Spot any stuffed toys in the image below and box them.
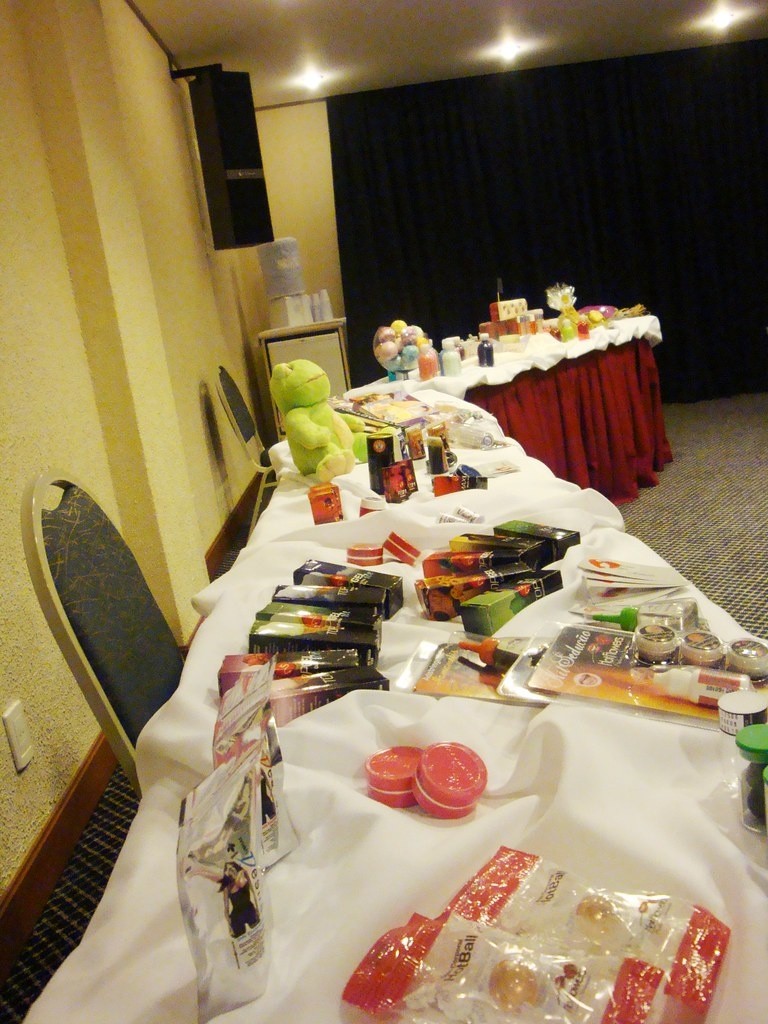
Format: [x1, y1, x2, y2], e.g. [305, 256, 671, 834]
[269, 359, 403, 484]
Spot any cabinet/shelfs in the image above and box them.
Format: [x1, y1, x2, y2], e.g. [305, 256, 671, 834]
[259, 321, 350, 442]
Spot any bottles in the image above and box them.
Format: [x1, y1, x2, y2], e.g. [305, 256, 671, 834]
[560, 319, 575, 343]
[360, 497, 383, 517]
[591, 597, 698, 633]
[418, 336, 465, 382]
[633, 626, 768, 690]
[477, 332, 495, 367]
[735, 724, 768, 838]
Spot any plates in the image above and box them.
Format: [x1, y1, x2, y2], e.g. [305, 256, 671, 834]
[578, 306, 616, 318]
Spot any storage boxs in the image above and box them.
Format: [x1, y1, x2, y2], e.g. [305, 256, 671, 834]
[218, 520, 580, 727]
[269, 295, 314, 329]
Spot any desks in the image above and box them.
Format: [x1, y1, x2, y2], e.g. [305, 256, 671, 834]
[23, 315, 768, 1024]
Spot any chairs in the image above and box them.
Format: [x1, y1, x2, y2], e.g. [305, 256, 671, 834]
[215, 366, 277, 543]
[23, 468, 185, 805]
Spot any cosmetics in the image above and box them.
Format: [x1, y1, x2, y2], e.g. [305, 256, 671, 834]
[308, 482, 343, 525]
[417, 332, 496, 382]
[360, 422, 488, 517]
[479, 297, 544, 344]
[413, 520, 581, 641]
[218, 558, 404, 726]
[363, 740, 489, 819]
[346, 530, 422, 567]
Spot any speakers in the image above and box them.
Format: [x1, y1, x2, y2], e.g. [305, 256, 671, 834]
[188, 70, 275, 250]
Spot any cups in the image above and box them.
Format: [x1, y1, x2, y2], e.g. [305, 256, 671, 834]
[366, 433, 394, 495]
[393, 460, 418, 492]
[381, 465, 410, 504]
